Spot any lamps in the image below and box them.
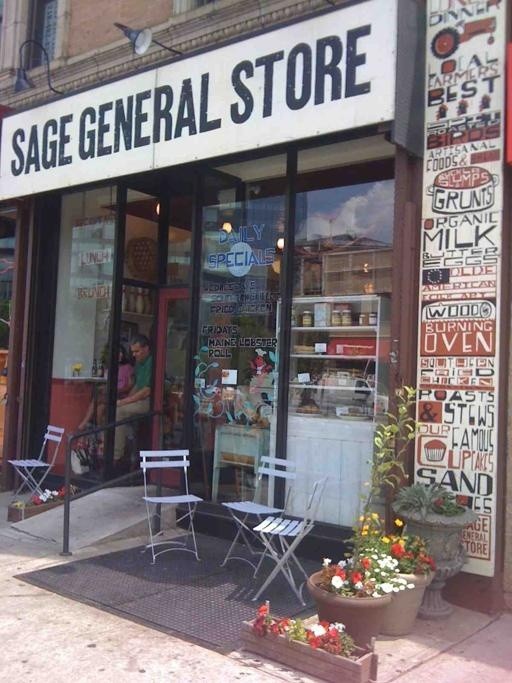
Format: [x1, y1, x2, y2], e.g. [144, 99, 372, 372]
[13, 39, 65, 96]
[112, 21, 183, 57]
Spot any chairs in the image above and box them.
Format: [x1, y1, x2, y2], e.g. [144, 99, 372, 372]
[139, 450, 204, 563]
[218, 456, 295, 579]
[251, 476, 328, 606]
[6, 424, 65, 502]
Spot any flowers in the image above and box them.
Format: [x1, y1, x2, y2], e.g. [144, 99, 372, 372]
[12, 483, 79, 508]
[321, 547, 415, 597]
[346, 513, 438, 574]
[249, 600, 357, 657]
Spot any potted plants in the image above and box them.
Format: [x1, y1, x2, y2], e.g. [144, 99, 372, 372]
[390, 480, 478, 620]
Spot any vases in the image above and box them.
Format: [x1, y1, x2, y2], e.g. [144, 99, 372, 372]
[380, 573, 434, 636]
[6, 501, 62, 522]
[308, 571, 392, 647]
[240, 614, 376, 682]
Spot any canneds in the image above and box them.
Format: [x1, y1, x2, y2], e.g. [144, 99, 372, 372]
[342, 310, 353, 326]
[358, 314, 366, 325]
[368, 313, 377, 326]
[331, 310, 342, 326]
[302, 310, 313, 327]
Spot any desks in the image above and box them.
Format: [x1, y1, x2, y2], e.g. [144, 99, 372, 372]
[211, 424, 270, 505]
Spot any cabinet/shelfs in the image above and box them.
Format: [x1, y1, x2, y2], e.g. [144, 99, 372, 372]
[292, 247, 392, 295]
[267, 296, 391, 531]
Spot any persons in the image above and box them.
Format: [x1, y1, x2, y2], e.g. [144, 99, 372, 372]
[74, 344, 134, 432]
[113, 333, 155, 461]
